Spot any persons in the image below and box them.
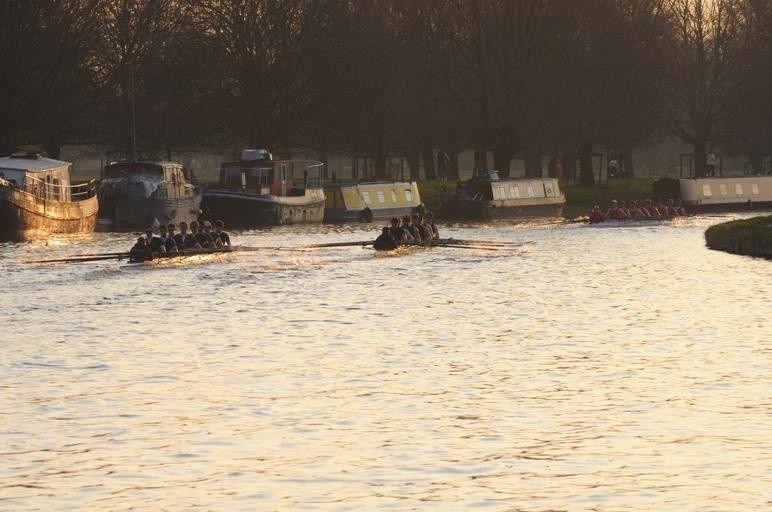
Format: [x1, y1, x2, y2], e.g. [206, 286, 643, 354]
[144, 220, 230, 254]
[390, 212, 439, 245]
[604, 199, 684, 219]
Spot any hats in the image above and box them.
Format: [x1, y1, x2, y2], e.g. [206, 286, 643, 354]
[145, 226, 153, 232]
[204, 221, 211, 226]
[215, 222, 223, 226]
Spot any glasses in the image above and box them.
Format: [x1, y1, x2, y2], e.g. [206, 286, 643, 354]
[168, 229, 173, 231]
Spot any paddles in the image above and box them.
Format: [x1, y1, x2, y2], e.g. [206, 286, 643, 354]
[22, 252, 129, 264]
[221, 239, 376, 252]
[412, 237, 514, 251]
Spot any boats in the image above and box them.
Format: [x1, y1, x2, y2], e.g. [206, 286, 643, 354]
[200, 148, 328, 225]
[0, 145, 100, 247]
[119, 249, 233, 268]
[578, 216, 683, 228]
[324, 180, 434, 222]
[94, 155, 203, 232]
[372, 234, 440, 258]
[447, 170, 567, 221]
